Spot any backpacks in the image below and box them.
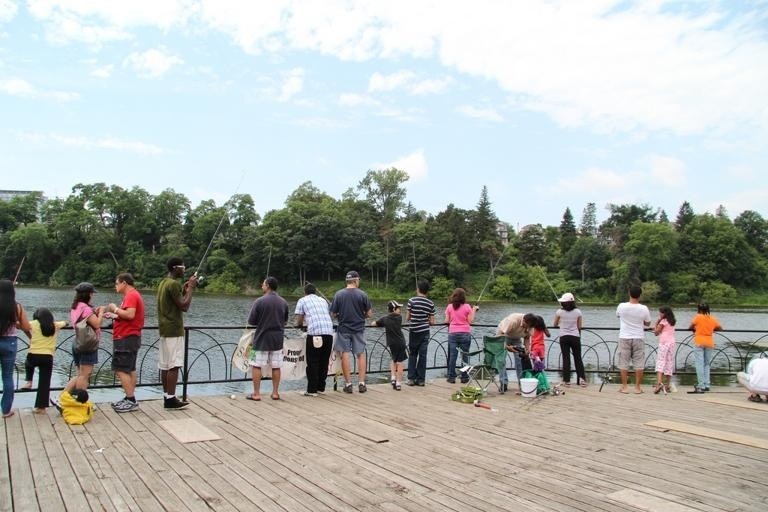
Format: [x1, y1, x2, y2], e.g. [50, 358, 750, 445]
[72, 305, 99, 355]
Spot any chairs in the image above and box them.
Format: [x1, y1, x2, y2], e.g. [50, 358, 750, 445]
[457, 335, 507, 397]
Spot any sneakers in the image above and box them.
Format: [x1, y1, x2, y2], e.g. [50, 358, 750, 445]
[1, 410, 15, 417]
[21, 382, 32, 389]
[653, 383, 672, 394]
[343, 383, 352, 394]
[446, 378, 455, 383]
[580, 381, 587, 387]
[73, 280, 99, 295]
[303, 390, 317, 397]
[317, 388, 327, 395]
[357, 383, 367, 392]
[694, 386, 710, 394]
[748, 395, 762, 402]
[390, 379, 402, 391]
[564, 382, 571, 388]
[163, 396, 190, 409]
[405, 380, 424, 387]
[32, 407, 48, 415]
[462, 375, 469, 383]
[110, 398, 139, 413]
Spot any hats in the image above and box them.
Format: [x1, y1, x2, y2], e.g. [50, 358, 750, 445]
[389, 301, 403, 310]
[557, 293, 575, 303]
[345, 271, 360, 281]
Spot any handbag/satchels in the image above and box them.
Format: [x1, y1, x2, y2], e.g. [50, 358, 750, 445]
[519, 368, 551, 395]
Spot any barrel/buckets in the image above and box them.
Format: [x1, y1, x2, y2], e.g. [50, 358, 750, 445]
[519, 378, 539, 398]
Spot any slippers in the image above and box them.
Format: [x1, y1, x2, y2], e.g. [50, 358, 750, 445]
[270, 393, 279, 400]
[246, 394, 260, 401]
[619, 387, 644, 394]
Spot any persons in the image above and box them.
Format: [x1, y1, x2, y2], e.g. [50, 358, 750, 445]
[0, 258, 200, 418]
[495, 285, 768, 403]
[246, 271, 479, 401]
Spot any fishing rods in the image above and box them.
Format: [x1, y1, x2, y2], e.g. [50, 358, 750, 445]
[194, 170, 244, 278]
[475, 241, 510, 309]
[12, 256, 26, 287]
[305, 281, 332, 305]
[535, 262, 562, 309]
[413, 240, 418, 289]
